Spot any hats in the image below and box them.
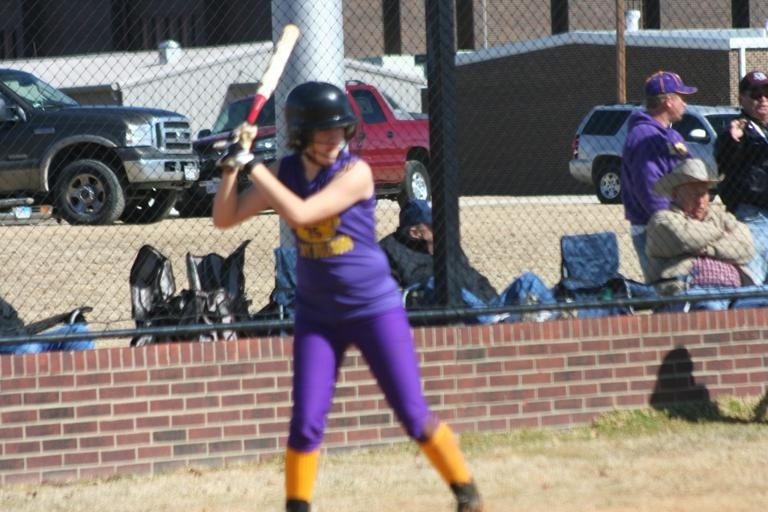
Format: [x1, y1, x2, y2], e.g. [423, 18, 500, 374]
[646, 72, 698, 95]
[654, 158, 724, 196]
[737, 70, 768, 90]
[400, 201, 432, 227]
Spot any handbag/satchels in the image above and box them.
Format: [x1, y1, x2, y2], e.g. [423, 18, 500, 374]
[130, 287, 237, 345]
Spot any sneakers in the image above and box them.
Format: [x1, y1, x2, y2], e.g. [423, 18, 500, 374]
[459, 493, 483, 512]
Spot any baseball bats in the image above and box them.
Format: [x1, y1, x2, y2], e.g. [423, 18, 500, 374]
[223, 25, 300, 169]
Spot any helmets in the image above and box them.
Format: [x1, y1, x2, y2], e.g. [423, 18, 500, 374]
[285, 79, 358, 149]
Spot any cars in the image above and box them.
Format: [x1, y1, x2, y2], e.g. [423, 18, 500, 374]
[175, 83, 432, 208]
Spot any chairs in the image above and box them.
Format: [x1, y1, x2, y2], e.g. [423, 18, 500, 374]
[548, 234, 659, 318]
[129, 240, 258, 342]
[0, 299, 95, 353]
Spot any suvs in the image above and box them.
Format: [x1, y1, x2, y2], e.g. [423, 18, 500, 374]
[1, 68, 202, 225]
[569, 102, 743, 204]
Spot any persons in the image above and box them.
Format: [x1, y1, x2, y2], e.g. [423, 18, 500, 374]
[212, 82, 486, 512]
[619, 70, 698, 272]
[713, 71, 768, 283]
[644, 158, 768, 312]
[378, 200, 497, 302]
